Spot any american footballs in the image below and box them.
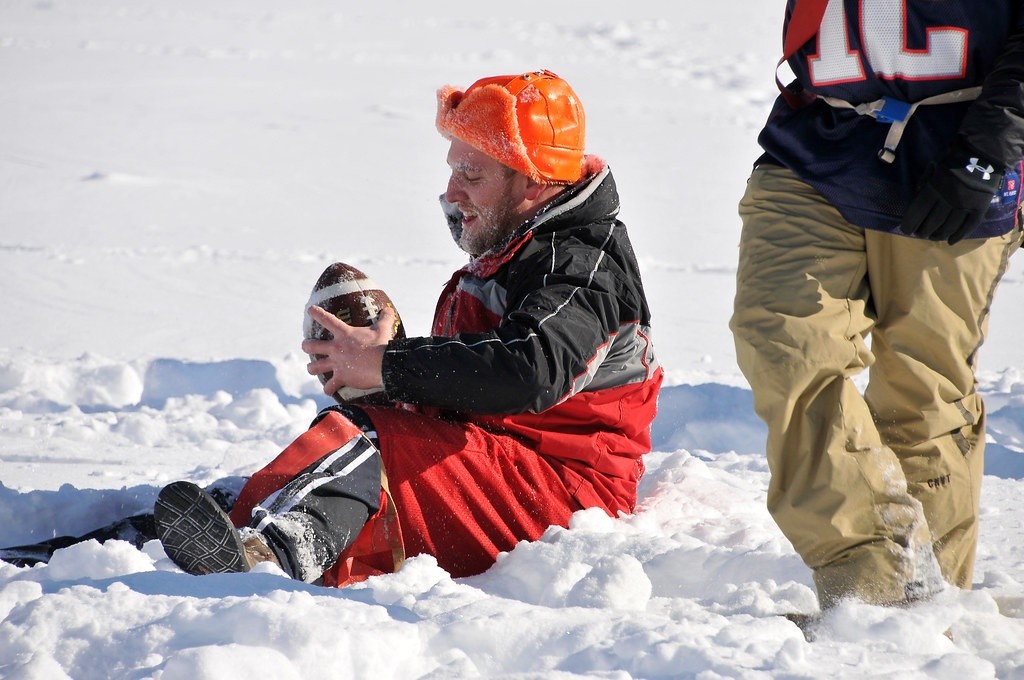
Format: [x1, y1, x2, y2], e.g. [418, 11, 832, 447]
[302, 262, 407, 404]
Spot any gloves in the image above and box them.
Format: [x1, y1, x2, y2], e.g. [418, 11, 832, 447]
[900, 138, 1005, 245]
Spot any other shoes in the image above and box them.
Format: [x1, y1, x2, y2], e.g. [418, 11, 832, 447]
[153, 480, 281, 577]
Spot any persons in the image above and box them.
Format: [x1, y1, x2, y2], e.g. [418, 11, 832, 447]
[732, 0, 1023, 635]
[0, 70, 663, 590]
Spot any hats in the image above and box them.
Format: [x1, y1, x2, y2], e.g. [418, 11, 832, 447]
[435, 70, 585, 185]
